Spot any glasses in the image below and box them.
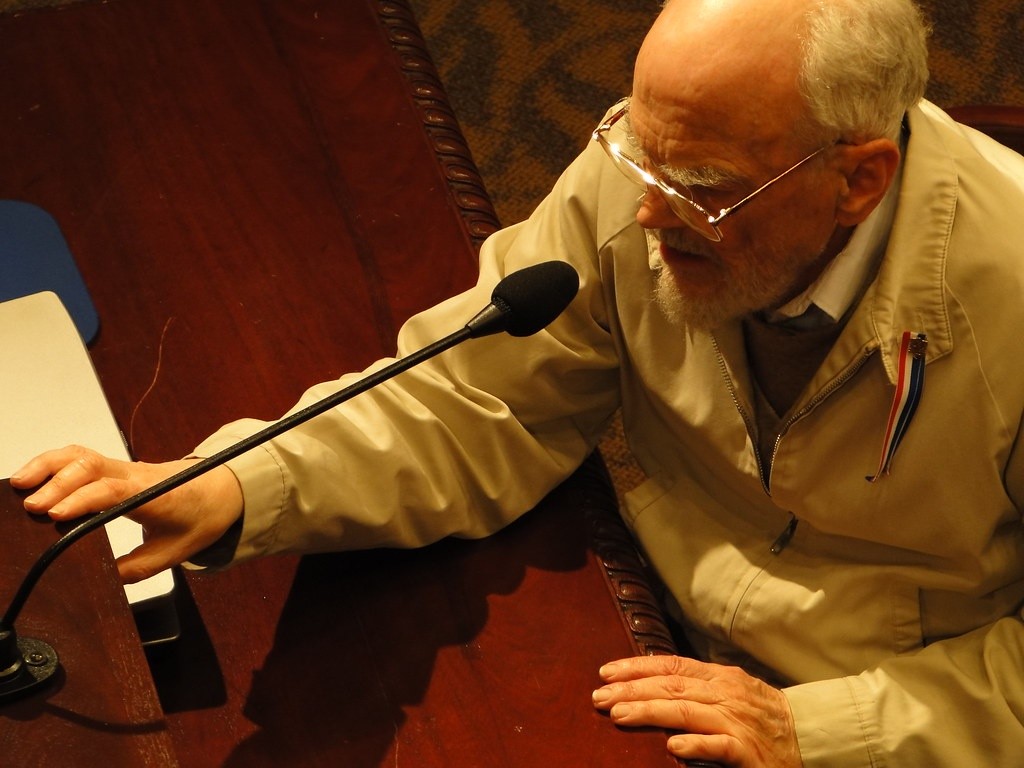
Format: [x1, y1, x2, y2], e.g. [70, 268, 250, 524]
[592, 101, 857, 242]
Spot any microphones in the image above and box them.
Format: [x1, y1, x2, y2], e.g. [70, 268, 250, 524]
[0, 259, 580, 708]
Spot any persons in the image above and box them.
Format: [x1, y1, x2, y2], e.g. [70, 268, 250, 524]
[10, 0, 1023, 768]
